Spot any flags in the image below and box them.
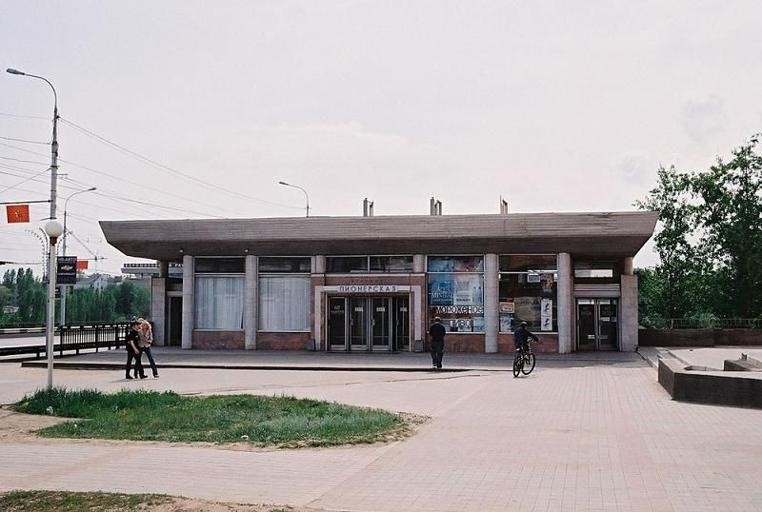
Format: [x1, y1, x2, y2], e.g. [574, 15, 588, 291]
[75, 261, 88, 270]
[6, 205, 30, 223]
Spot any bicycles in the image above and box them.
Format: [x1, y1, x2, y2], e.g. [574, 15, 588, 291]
[513, 338, 538, 377]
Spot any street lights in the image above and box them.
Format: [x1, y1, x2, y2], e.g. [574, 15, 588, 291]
[45, 219, 63, 391]
[5, 67, 59, 344]
[60, 185, 98, 328]
[278, 180, 310, 217]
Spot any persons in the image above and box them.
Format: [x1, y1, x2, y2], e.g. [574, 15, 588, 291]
[429, 316, 446, 368]
[134, 320, 160, 378]
[512, 321, 542, 371]
[125, 319, 149, 379]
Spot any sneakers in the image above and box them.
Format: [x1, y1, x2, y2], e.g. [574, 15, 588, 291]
[127, 373, 160, 379]
